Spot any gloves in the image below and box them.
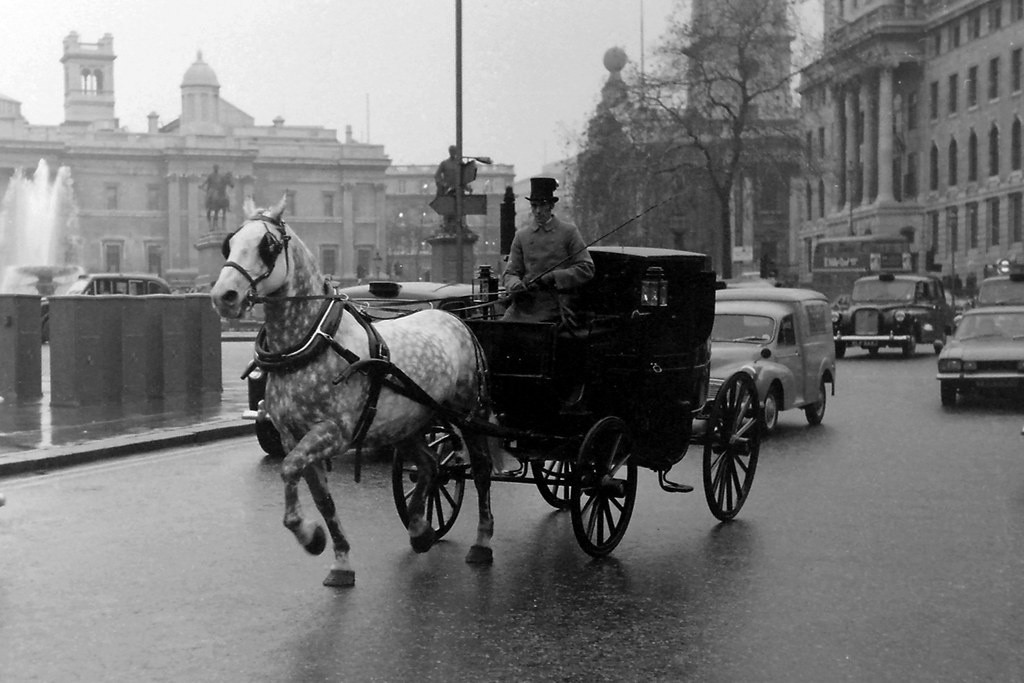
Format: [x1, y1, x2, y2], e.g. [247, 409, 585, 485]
[511, 282, 526, 295]
[537, 272, 555, 290]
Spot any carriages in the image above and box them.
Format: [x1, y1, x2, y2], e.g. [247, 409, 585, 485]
[209, 188, 763, 589]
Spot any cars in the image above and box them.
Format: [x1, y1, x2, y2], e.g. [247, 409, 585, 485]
[939, 273, 1024, 407]
[832, 272, 950, 360]
[0, 261, 394, 345]
[688, 285, 838, 441]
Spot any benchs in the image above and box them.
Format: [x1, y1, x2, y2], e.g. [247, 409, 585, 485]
[505, 269, 624, 356]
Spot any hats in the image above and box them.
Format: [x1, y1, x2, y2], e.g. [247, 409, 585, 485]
[525, 177, 559, 203]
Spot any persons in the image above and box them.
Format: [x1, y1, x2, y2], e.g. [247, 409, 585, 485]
[435, 146, 469, 231]
[200, 164, 232, 211]
[503, 177, 594, 321]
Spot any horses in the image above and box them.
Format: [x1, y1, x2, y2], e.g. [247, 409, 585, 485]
[209, 193, 496, 588]
